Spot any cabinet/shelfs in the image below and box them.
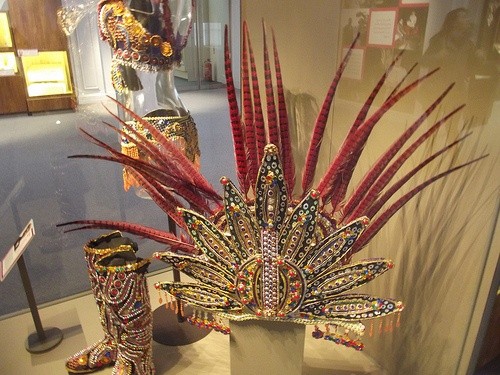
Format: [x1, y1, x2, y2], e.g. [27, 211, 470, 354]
[0, 0, 78, 116]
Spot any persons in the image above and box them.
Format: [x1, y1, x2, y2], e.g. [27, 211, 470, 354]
[342, 17, 354, 47]
[409, 10, 417, 25]
[421, 7, 476, 141]
[356, 11, 368, 47]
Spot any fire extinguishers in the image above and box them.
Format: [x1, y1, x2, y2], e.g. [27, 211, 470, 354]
[204, 59, 212, 81]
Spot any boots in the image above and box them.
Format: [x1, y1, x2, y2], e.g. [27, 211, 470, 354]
[96, 250, 159, 375]
[65, 230, 139, 374]
